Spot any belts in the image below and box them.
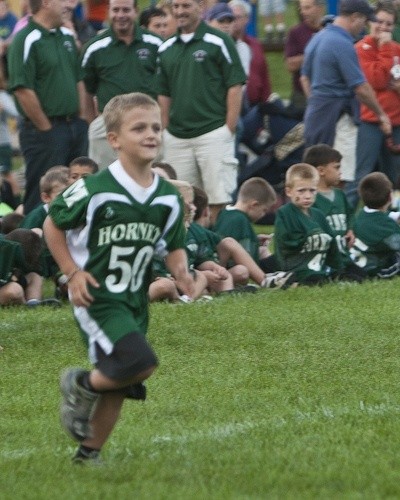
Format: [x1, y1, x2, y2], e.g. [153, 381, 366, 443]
[24, 114, 80, 122]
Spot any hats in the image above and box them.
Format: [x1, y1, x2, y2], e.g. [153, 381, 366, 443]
[321, 15, 335, 25]
[339, 0, 376, 22]
[206, 2, 235, 21]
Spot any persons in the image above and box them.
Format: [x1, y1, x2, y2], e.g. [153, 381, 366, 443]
[0, 0, 400, 310]
[42, 91, 197, 467]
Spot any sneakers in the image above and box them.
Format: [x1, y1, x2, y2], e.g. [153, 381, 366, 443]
[59, 367, 101, 442]
[71, 456, 97, 466]
[245, 283, 260, 294]
[261, 270, 298, 291]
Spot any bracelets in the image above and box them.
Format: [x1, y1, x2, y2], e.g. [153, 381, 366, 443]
[67, 269, 79, 282]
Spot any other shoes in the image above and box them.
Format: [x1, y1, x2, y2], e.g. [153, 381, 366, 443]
[178, 294, 212, 304]
[25, 298, 62, 309]
